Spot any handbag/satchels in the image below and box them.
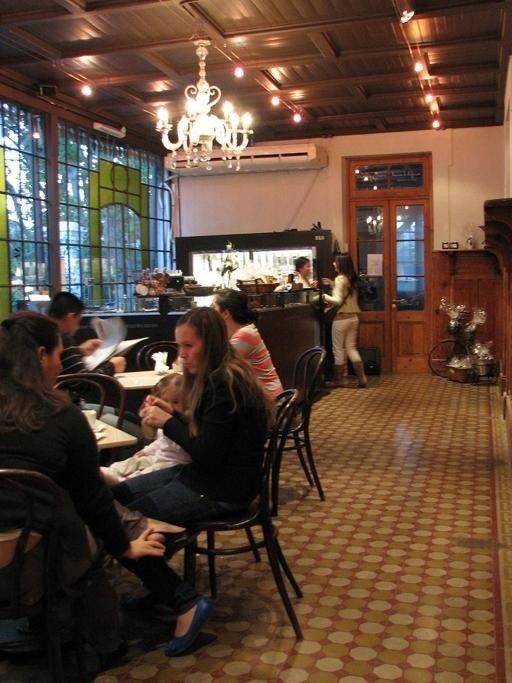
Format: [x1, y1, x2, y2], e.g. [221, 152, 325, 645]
[317, 301, 342, 321]
[40, 566, 129, 683]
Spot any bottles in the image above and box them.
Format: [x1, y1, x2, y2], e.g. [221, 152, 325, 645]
[208, 254, 307, 270]
[123, 294, 129, 312]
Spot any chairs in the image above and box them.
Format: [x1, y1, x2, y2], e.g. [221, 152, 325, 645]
[0, 469, 107, 683]
[184, 388, 304, 641]
[53, 373, 125, 430]
[266, 348, 329, 501]
[136, 341, 180, 371]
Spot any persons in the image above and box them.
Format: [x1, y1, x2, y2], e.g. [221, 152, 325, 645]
[0, 310, 168, 676]
[106, 305, 270, 660]
[276, 254, 318, 291]
[317, 251, 367, 388]
[97, 370, 192, 487]
[210, 288, 291, 511]
[45, 290, 141, 424]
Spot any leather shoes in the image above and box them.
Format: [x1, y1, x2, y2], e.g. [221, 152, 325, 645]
[125, 580, 166, 608]
[164, 595, 210, 657]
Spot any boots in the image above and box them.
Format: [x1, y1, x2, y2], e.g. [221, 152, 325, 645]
[351, 361, 368, 388]
[323, 363, 349, 388]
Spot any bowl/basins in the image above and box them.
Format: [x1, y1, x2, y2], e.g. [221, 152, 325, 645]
[184, 287, 215, 294]
[238, 283, 280, 292]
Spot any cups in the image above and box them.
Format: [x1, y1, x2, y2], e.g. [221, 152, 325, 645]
[83, 410, 97, 426]
[287, 273, 303, 288]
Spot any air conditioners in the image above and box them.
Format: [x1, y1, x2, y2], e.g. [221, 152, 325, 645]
[162, 142, 331, 177]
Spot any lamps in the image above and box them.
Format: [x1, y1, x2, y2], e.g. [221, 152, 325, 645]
[154, 34, 255, 171]
